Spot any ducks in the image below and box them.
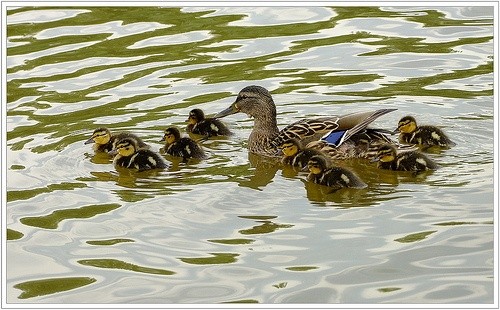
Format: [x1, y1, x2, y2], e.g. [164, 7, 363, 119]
[391, 116, 456, 148]
[83, 127, 150, 154]
[276, 140, 329, 169]
[300, 157, 369, 189]
[374, 147, 441, 172]
[107, 138, 174, 172]
[161, 127, 206, 159]
[213, 84, 400, 160]
[185, 109, 234, 143]
